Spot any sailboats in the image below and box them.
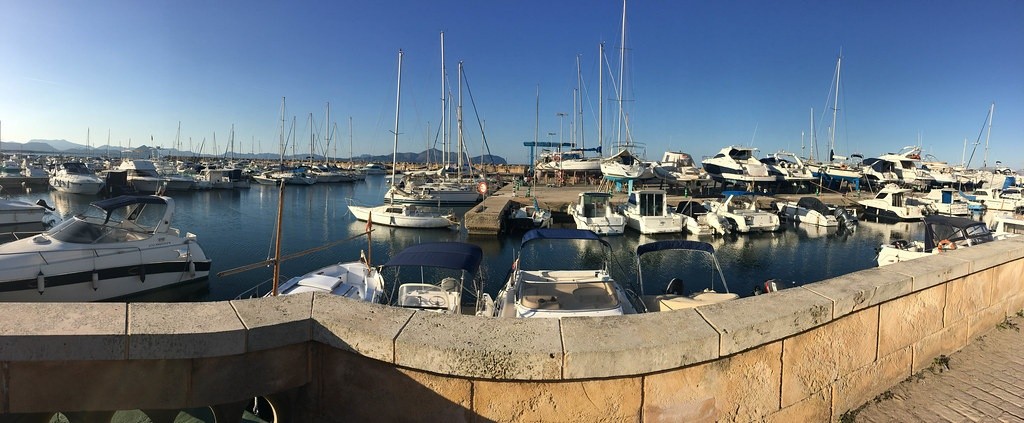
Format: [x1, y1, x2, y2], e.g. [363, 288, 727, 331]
[800, 47, 864, 180]
[383, 29, 506, 207]
[535, 0, 649, 176]
[344, 49, 464, 230]
[252, 95, 367, 187]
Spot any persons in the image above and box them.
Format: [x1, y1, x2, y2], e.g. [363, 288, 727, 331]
[524, 171, 539, 185]
[754, 184, 772, 197]
[971, 200, 987, 221]
[845, 181, 854, 197]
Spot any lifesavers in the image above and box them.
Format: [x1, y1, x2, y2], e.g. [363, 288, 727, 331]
[553, 155, 560, 162]
[937, 239, 956, 252]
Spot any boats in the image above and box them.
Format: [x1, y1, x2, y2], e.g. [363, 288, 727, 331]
[617, 188, 683, 235]
[359, 163, 387, 175]
[0, 199, 56, 236]
[0, 194, 218, 303]
[258, 257, 386, 306]
[624, 239, 740, 313]
[0, 151, 246, 197]
[367, 241, 493, 319]
[493, 228, 638, 318]
[568, 190, 627, 236]
[648, 127, 1024, 271]
[669, 200, 731, 237]
[505, 198, 553, 231]
[701, 190, 781, 234]
[775, 196, 860, 236]
